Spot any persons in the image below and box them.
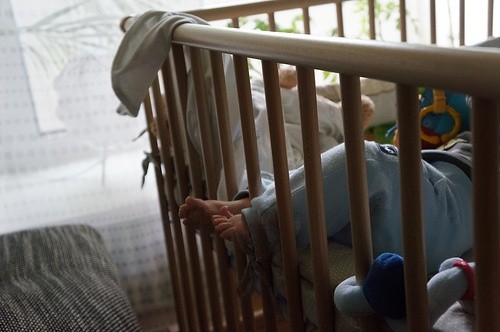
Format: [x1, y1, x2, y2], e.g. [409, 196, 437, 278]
[178, 131, 473, 274]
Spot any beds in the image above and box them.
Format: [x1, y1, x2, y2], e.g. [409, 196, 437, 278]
[120, 0, 500, 332]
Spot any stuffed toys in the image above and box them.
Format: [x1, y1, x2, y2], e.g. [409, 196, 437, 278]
[334, 252, 476, 332]
[149, 66, 374, 136]
